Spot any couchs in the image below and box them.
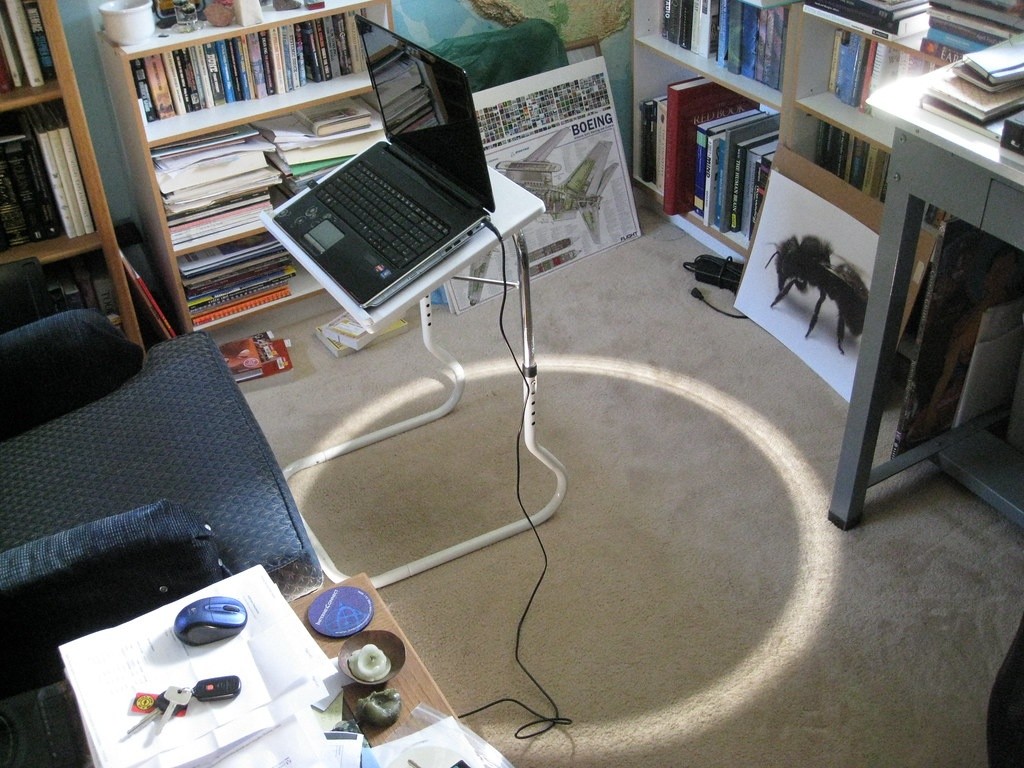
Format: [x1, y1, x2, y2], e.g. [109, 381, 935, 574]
[1, 310, 323, 703]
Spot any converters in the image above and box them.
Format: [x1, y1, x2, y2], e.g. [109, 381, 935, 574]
[694, 256, 744, 291]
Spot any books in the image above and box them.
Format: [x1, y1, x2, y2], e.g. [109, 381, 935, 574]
[0, 0, 116, 319]
[314, 311, 410, 358]
[119, 248, 177, 340]
[637, 0, 1024, 261]
[218, 331, 295, 392]
[129, 8, 367, 328]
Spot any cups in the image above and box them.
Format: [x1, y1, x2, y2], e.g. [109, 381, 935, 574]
[99, 0, 156, 46]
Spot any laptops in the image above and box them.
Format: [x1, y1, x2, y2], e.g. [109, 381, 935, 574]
[273, 13, 495, 309]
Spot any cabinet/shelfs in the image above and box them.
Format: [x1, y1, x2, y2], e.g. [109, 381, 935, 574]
[0, 0, 148, 366]
[633, 0, 1024, 267]
[94, 0, 450, 338]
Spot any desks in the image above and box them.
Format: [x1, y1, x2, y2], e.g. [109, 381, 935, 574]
[828, 60, 1024, 541]
[292, 577, 458, 747]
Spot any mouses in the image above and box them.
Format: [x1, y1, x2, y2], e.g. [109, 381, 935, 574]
[173, 595, 247, 647]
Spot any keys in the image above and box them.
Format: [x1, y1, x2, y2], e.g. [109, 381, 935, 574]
[127, 675, 241, 737]
